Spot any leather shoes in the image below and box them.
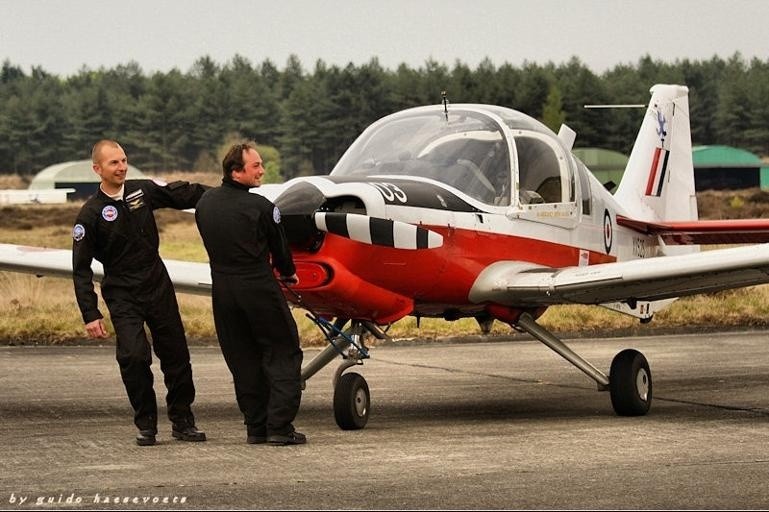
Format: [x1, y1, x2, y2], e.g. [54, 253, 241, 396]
[171, 425, 207, 442]
[267, 429, 307, 444]
[135, 428, 157, 446]
[247, 432, 267, 444]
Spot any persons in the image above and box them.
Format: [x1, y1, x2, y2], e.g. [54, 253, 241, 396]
[195, 139, 306, 447]
[72, 138, 219, 446]
